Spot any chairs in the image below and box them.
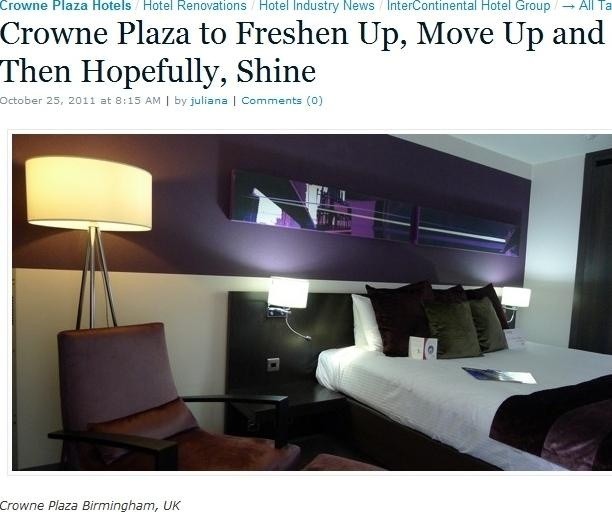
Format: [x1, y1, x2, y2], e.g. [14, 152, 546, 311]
[47, 322, 301, 471]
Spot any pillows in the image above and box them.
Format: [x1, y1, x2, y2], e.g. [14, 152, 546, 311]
[351, 279, 511, 359]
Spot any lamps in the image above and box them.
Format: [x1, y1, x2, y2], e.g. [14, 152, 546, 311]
[501, 286, 531, 323]
[266, 276, 312, 342]
[25, 157, 153, 330]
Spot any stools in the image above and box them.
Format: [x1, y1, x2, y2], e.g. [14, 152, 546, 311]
[302, 452, 389, 471]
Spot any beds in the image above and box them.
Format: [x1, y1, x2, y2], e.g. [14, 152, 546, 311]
[316, 339, 612, 471]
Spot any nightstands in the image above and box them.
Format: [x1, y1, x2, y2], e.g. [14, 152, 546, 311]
[229, 381, 354, 463]
